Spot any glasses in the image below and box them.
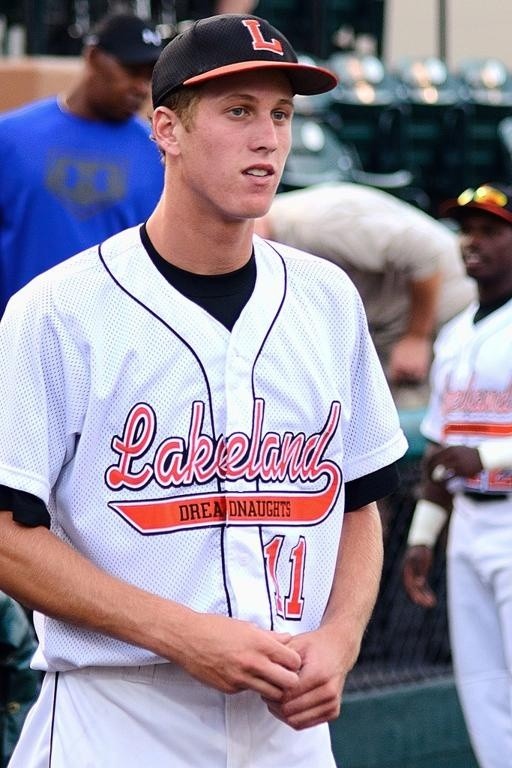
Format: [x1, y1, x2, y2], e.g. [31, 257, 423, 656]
[455, 186, 508, 208]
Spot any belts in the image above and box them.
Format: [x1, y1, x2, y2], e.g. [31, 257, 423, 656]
[463, 491, 507, 501]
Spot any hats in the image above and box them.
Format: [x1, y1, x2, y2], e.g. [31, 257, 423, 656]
[152, 14, 338, 110]
[89, 13, 163, 76]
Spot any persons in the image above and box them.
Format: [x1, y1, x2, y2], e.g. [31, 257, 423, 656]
[0, 9, 412, 765]
[251, 180, 479, 507]
[401, 171, 512, 767]
[0, 10, 163, 322]
